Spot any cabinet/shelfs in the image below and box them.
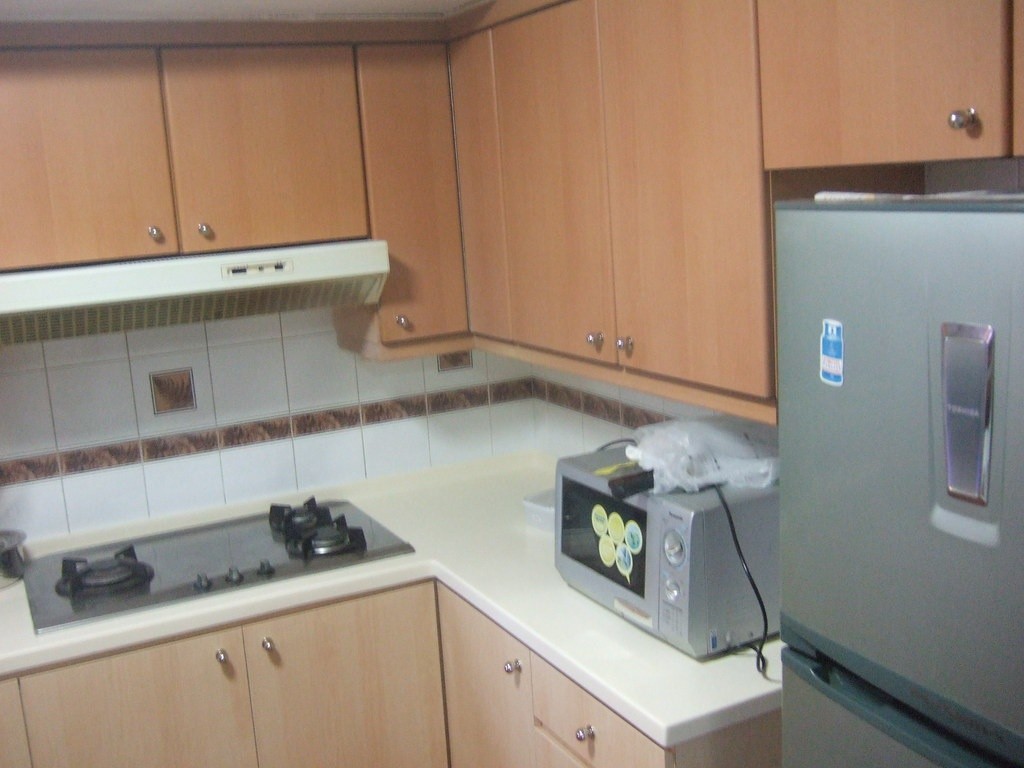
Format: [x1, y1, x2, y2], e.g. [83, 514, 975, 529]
[18, 577, 450, 768]
[445, 0, 779, 428]
[756, 0, 1024, 172]
[434, 578, 781, 768]
[0, 20, 371, 272]
[333, 20, 472, 364]
